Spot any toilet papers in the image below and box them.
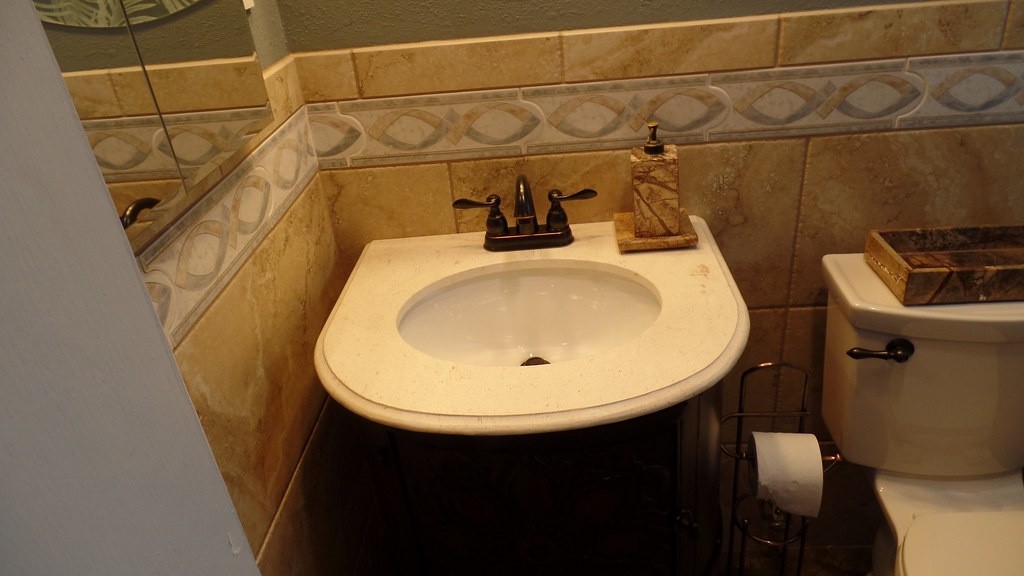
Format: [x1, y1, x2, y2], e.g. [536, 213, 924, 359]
[745, 430, 826, 520]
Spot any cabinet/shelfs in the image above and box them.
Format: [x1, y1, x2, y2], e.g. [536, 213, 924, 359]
[340, 382, 726, 575]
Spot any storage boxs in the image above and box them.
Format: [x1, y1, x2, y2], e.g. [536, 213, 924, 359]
[864, 224, 1024, 305]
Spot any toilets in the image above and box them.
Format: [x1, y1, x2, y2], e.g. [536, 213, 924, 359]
[817, 249, 1024, 576]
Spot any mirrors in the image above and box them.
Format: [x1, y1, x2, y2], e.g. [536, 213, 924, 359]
[33, 0, 275, 242]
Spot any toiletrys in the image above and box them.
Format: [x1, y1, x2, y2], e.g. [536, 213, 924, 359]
[629, 120, 681, 239]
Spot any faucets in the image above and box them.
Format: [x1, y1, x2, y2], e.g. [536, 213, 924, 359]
[513, 172, 538, 236]
[120, 197, 160, 231]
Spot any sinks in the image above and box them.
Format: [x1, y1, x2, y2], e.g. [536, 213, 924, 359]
[313, 247, 751, 437]
